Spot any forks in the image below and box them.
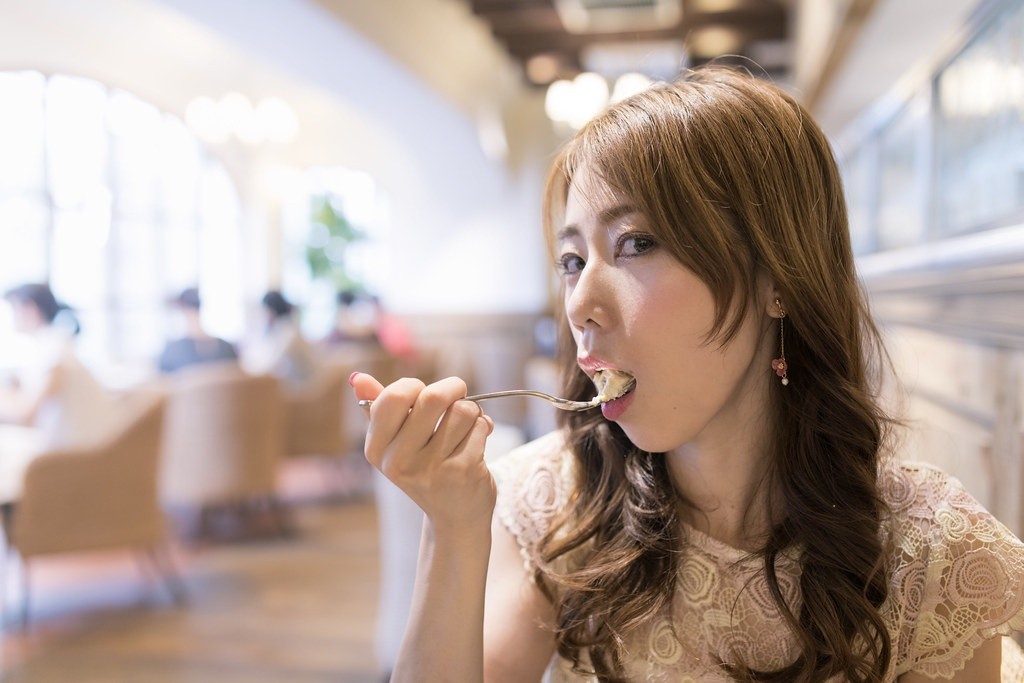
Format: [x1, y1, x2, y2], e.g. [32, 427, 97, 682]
[358, 372, 634, 412]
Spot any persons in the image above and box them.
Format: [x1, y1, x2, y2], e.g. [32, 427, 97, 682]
[348, 68, 1024, 683]
[322, 288, 379, 347]
[0, 284, 96, 436]
[257, 292, 314, 398]
[365, 294, 416, 352]
[149, 286, 239, 377]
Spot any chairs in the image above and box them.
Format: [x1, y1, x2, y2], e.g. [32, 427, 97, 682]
[0, 349, 442, 639]
